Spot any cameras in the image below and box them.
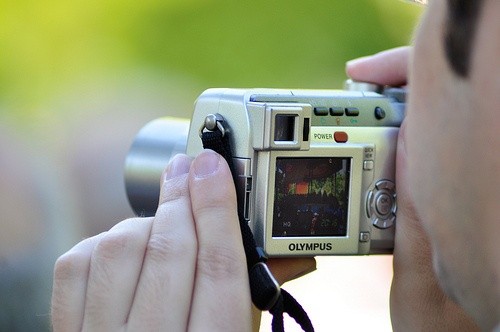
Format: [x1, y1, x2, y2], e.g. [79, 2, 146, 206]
[125, 78, 408, 258]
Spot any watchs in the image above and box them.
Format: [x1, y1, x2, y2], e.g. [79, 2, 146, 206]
[49, 1, 499, 332]
